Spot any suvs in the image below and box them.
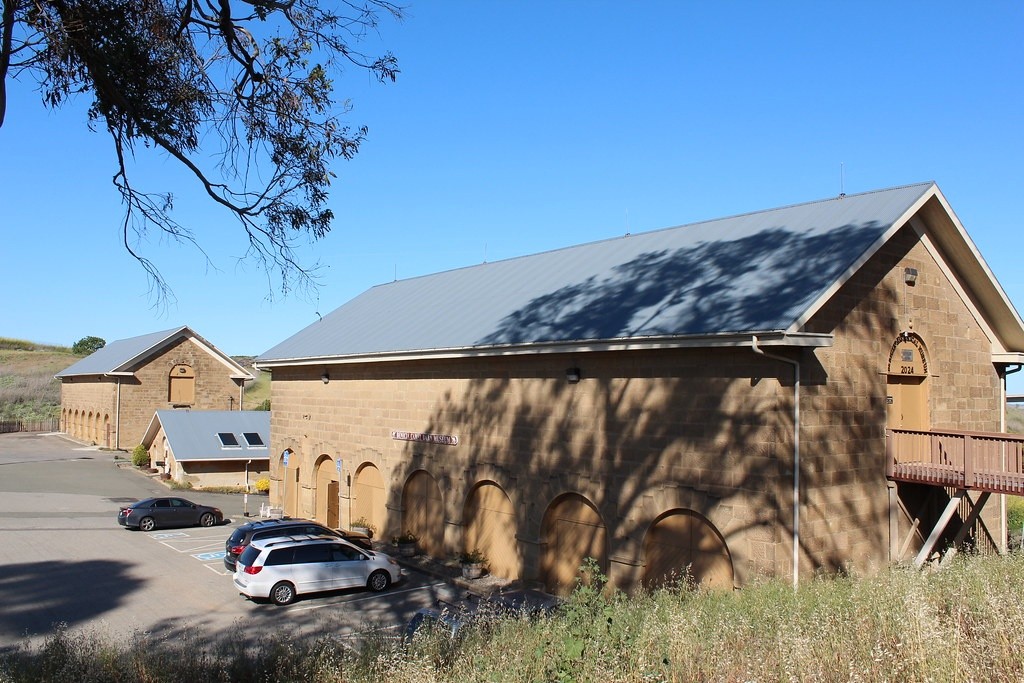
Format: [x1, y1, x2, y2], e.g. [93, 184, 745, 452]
[232, 535, 402, 606]
[223, 517, 371, 574]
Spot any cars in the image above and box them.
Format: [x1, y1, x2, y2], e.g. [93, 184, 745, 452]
[117, 496, 223, 532]
